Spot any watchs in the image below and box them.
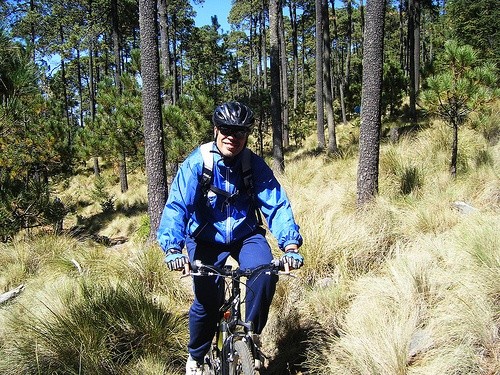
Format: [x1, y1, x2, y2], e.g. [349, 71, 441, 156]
[286, 249, 299, 253]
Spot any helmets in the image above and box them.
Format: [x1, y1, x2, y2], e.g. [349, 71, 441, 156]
[213, 100, 256, 132]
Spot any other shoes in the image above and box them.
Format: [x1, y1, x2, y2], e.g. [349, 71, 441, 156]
[184, 360, 200, 375]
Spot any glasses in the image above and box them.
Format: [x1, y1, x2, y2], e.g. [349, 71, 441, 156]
[217, 125, 245, 139]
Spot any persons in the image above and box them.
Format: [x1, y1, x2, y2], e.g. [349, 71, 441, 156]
[157, 102, 304, 375]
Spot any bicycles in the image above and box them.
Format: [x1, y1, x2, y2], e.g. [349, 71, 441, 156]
[177, 258, 297, 375]
